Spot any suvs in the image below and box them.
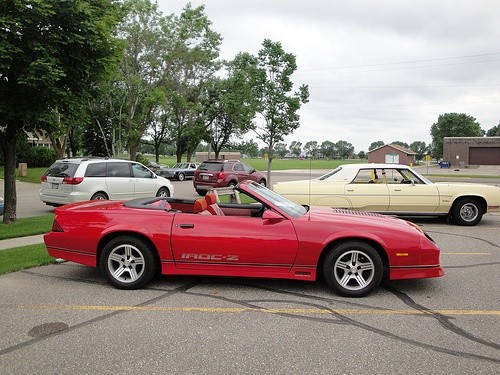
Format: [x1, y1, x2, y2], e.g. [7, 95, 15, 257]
[192, 159, 267, 197]
[38, 156, 174, 208]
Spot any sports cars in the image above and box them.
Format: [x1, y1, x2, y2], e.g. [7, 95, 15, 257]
[43, 180, 444, 298]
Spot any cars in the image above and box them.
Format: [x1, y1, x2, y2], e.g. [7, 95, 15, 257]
[271, 163, 500, 226]
[148, 161, 201, 181]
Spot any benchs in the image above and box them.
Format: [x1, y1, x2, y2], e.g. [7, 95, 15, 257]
[150, 200, 182, 212]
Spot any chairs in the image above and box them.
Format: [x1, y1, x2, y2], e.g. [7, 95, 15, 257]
[205, 193, 225, 216]
[194, 199, 213, 215]
